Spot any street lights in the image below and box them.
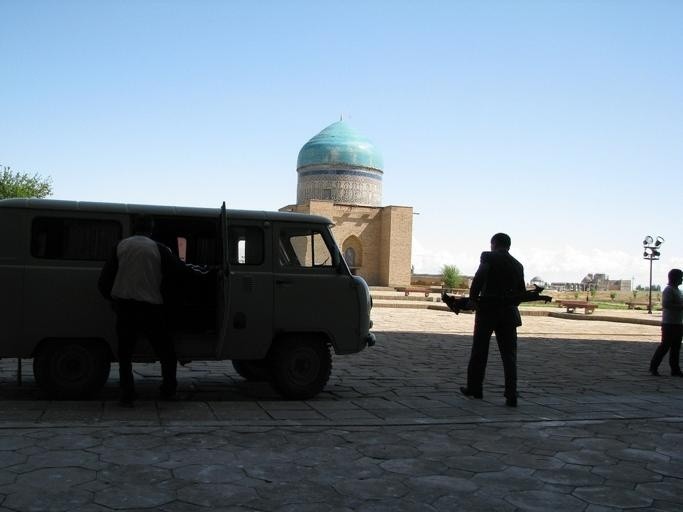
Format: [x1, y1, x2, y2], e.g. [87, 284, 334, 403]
[643, 237, 664, 314]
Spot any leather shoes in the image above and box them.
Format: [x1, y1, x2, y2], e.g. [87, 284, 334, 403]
[506, 399, 517, 407]
[460, 386, 482, 398]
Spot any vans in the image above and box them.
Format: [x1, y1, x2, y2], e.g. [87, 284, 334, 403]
[0, 198, 375, 401]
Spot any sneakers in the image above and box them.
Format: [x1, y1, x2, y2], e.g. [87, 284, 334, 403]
[650, 369, 658, 375]
[672, 371, 683, 376]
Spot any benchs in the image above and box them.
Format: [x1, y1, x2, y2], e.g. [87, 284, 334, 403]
[625, 302, 655, 310]
[394, 286, 432, 297]
[444, 288, 468, 295]
[556, 300, 598, 315]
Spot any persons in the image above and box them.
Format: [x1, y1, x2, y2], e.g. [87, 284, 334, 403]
[650, 268, 683, 377]
[460, 232, 527, 407]
[98, 214, 187, 408]
